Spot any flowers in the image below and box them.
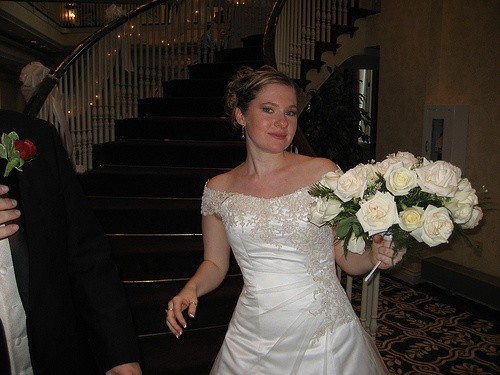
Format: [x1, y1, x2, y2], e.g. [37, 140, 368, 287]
[307, 151, 487, 281]
[0, 131, 39, 185]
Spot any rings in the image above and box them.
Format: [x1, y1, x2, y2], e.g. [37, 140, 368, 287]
[190, 301, 198, 307]
[168, 308, 174, 312]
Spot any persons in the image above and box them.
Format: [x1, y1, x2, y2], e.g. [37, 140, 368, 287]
[0, 107, 145, 375]
[165, 64, 407, 375]
